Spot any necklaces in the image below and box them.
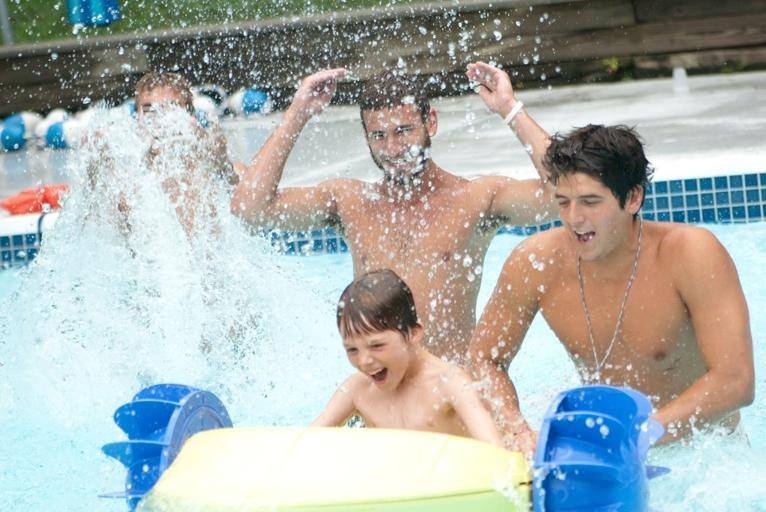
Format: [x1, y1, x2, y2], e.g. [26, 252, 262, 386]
[577, 215, 643, 373]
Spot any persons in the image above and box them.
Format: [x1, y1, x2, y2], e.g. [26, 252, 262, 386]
[79, 69, 242, 263]
[306, 267, 502, 450]
[231, 60, 562, 368]
[468, 125, 756, 460]
